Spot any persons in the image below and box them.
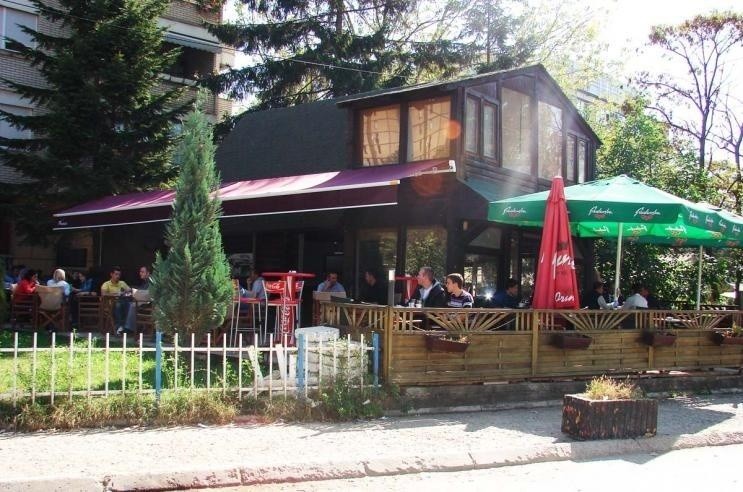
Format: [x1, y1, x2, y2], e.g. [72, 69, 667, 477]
[397, 265, 446, 307]
[483, 279, 518, 307]
[358, 268, 387, 305]
[443, 273, 475, 308]
[316, 270, 346, 292]
[2, 263, 271, 345]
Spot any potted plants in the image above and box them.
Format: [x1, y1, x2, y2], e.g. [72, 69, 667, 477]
[643, 331, 676, 346]
[423, 334, 473, 352]
[552, 371, 659, 442]
[713, 321, 743, 345]
[554, 330, 593, 349]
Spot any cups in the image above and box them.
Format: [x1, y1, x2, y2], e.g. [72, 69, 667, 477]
[408, 299, 422, 308]
[119, 288, 136, 297]
[607, 301, 621, 310]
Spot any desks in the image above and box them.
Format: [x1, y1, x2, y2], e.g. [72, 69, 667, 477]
[364, 302, 700, 329]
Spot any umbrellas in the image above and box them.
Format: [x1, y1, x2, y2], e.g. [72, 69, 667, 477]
[487, 175, 743, 311]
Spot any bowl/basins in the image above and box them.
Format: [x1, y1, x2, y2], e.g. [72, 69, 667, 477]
[666, 317, 673, 320]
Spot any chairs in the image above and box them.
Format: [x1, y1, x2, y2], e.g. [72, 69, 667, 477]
[0, 271, 316, 348]
